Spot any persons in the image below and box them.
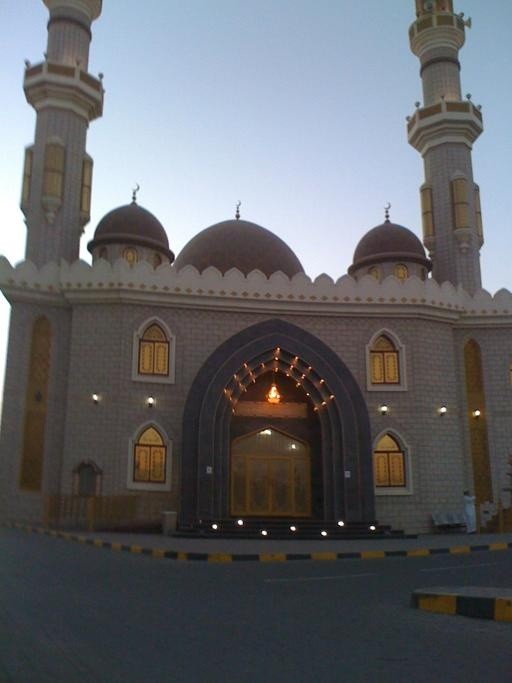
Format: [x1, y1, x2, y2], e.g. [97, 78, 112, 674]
[462, 487, 477, 535]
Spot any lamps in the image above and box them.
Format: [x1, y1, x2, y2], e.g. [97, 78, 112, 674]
[92, 392, 99, 405]
[380, 403, 388, 416]
[146, 396, 154, 407]
[439, 405, 447, 416]
[474, 408, 481, 419]
[266, 371, 280, 405]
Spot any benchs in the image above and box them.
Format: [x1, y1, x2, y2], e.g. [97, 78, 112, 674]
[430, 511, 467, 534]
